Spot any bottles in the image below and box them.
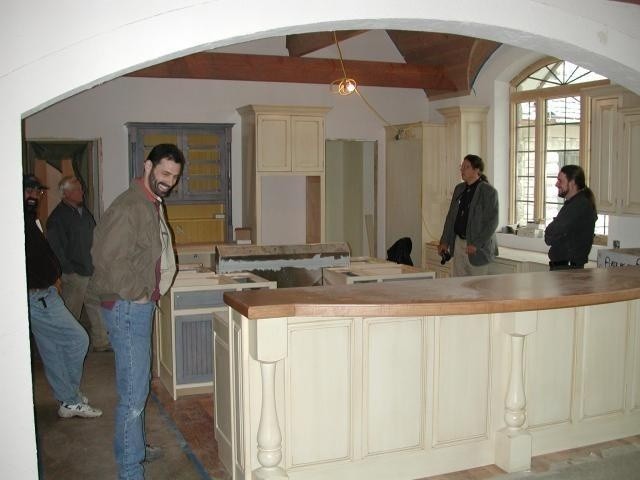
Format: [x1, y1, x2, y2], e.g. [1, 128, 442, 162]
[538, 219, 546, 232]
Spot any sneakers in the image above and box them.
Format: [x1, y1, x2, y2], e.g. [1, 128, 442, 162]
[59, 396, 102, 417]
[92, 342, 112, 353]
[145, 445, 163, 462]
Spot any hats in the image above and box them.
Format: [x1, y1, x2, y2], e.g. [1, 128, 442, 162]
[23, 175, 49, 189]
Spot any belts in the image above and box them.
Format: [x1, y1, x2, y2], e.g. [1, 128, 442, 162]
[548, 260, 577, 266]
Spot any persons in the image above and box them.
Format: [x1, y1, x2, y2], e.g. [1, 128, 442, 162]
[45, 174, 97, 318]
[90, 143, 186, 480]
[23, 172, 101, 417]
[544, 164, 598, 270]
[437, 154, 499, 275]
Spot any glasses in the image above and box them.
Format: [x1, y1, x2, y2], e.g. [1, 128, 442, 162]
[25, 188, 43, 195]
[459, 164, 471, 169]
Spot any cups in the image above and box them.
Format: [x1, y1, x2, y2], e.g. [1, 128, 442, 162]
[613, 240, 621, 249]
[535, 228, 543, 238]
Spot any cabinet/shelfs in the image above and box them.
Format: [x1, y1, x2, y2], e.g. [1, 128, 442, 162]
[121, 120, 236, 244]
[319, 261, 436, 285]
[578, 83, 639, 217]
[235, 101, 334, 246]
[154, 270, 279, 402]
[425, 239, 552, 280]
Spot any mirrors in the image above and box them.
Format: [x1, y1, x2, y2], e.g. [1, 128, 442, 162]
[324, 137, 379, 259]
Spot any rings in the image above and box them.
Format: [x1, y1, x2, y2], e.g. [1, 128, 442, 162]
[440, 247, 443, 251]
